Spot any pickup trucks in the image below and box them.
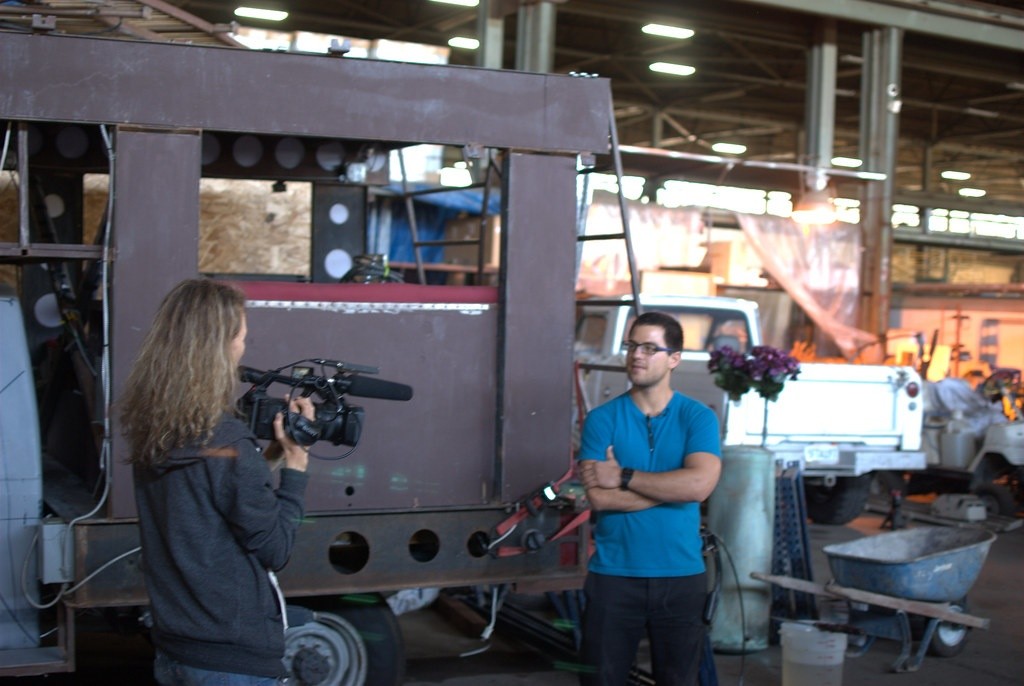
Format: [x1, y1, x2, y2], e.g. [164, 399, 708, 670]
[573, 292, 928, 526]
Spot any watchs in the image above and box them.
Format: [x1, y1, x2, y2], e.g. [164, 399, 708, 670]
[620, 467, 633, 488]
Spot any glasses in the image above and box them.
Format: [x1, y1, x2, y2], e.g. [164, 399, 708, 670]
[622, 339, 673, 355]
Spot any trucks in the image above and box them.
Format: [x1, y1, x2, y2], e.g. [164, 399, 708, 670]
[0, 31, 608, 686]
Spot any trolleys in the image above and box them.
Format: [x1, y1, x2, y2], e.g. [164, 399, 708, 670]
[748, 523, 999, 672]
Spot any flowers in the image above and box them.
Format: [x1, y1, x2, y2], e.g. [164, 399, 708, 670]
[703, 344, 801, 401]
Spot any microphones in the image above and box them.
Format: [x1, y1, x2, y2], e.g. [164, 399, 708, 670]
[328, 375, 412, 401]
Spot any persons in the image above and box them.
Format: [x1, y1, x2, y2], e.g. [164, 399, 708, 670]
[576, 312, 722, 685]
[114, 279, 314, 686]
